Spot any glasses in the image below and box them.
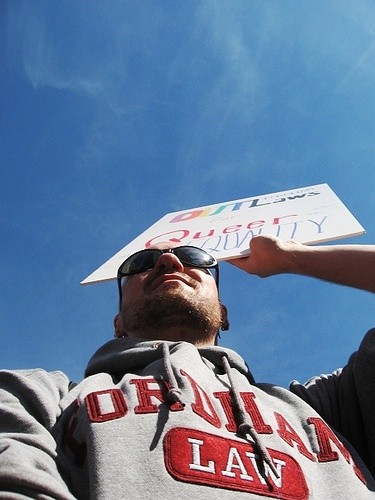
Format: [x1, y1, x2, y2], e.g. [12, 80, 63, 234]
[117, 244, 221, 306]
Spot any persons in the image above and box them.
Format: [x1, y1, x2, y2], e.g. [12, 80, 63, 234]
[0, 234, 375, 499]
